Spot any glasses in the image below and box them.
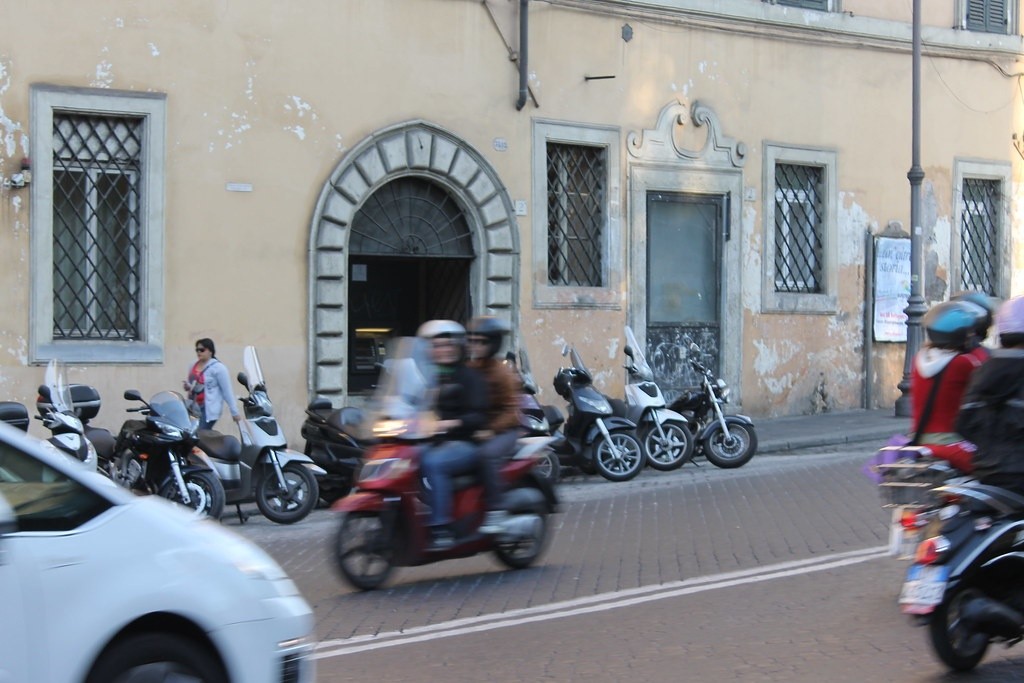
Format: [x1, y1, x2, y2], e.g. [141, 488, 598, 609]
[467, 338, 489, 345]
[195, 348, 205, 352]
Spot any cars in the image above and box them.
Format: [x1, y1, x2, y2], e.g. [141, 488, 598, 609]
[0, 407, 325, 683]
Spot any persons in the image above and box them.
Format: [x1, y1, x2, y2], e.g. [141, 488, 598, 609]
[181, 338, 241, 429]
[400, 316, 521, 551]
[908, 292, 996, 475]
[955, 294, 1024, 494]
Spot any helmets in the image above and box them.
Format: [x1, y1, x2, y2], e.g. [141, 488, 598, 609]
[416, 319, 467, 368]
[465, 318, 511, 358]
[950, 290, 994, 322]
[923, 300, 986, 348]
[997, 297, 1024, 338]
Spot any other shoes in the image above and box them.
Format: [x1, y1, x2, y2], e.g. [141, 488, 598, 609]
[424, 536, 454, 552]
[475, 509, 514, 533]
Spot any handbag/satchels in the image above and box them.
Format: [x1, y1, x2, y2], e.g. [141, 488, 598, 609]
[185, 398, 202, 418]
[865, 433, 916, 484]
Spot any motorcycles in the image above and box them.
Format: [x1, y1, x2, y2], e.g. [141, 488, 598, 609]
[870, 442, 1024, 671]
[330, 336, 563, 591]
[667, 343, 758, 469]
[302, 362, 444, 503]
[0, 383, 101, 487]
[499, 347, 574, 486]
[199, 345, 329, 525]
[601, 323, 696, 469]
[542, 340, 648, 483]
[64, 374, 227, 523]
